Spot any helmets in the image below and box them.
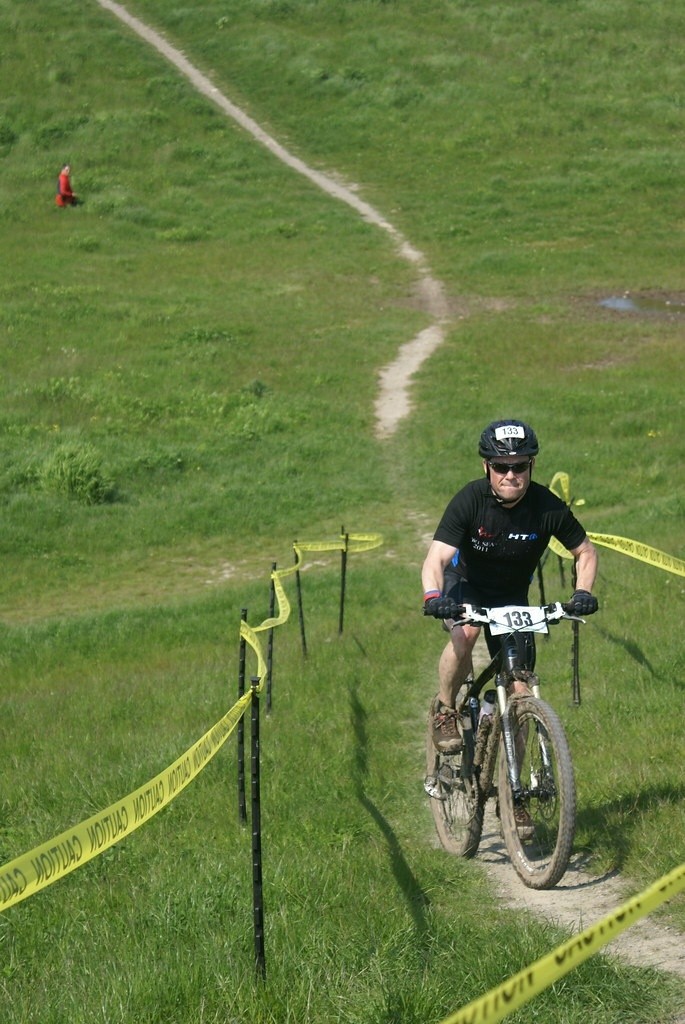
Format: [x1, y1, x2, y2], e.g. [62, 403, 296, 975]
[478, 419, 539, 457]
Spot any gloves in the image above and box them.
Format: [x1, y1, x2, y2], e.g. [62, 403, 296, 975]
[422, 589, 460, 620]
[570, 590, 599, 616]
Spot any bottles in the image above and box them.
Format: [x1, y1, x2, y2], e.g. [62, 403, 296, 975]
[478, 689, 499, 734]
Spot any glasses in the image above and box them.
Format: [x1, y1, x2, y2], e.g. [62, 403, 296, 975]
[487, 459, 532, 474]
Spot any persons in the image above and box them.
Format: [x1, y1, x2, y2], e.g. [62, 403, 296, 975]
[56, 163, 77, 208]
[421, 419, 598, 837]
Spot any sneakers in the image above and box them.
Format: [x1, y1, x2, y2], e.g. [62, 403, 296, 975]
[427, 691, 465, 757]
[494, 799, 535, 835]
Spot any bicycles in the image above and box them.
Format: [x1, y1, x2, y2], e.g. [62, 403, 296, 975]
[418, 592, 598, 891]
